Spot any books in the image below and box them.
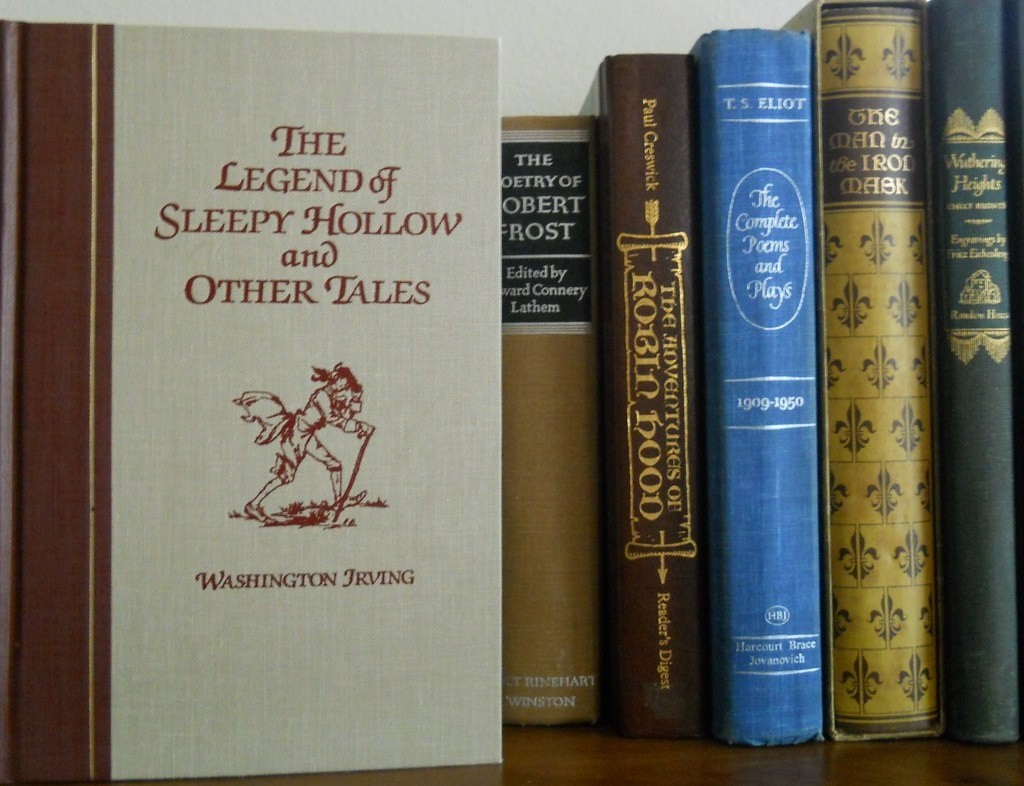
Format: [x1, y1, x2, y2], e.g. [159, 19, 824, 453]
[501, 1, 1022, 751]
[1, 19, 507, 786]
